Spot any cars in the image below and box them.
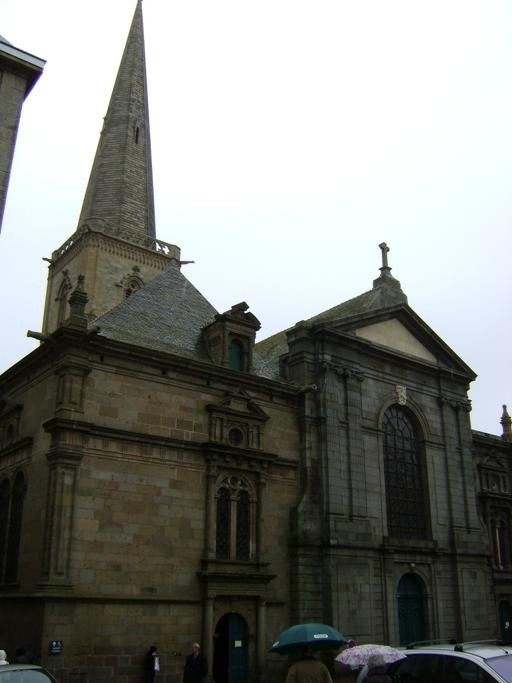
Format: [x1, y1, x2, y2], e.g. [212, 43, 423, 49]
[0, 658, 58, 682]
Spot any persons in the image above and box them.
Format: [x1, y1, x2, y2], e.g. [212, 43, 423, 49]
[143, 644, 160, 683]
[346, 639, 360, 680]
[0, 649, 10, 665]
[284, 644, 334, 682]
[364, 653, 393, 683]
[180, 641, 209, 683]
[14, 647, 41, 664]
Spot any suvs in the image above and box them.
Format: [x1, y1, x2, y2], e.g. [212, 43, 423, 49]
[356, 636, 512, 682]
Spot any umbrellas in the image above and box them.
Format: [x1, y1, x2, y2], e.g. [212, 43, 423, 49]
[268, 621, 348, 656]
[332, 643, 404, 665]
